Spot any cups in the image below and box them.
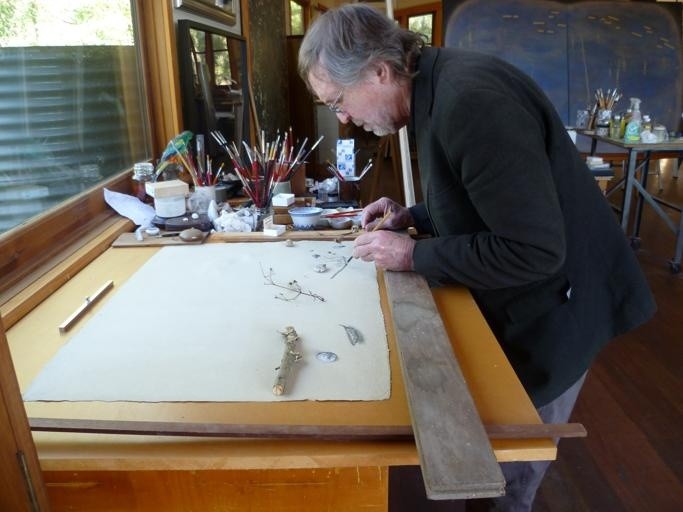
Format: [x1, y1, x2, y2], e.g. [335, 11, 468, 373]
[194, 185, 217, 216]
[596, 110, 612, 137]
[339, 176, 361, 201]
[652, 124, 669, 143]
[271, 181, 292, 196]
[253, 206, 274, 232]
[576, 110, 589, 130]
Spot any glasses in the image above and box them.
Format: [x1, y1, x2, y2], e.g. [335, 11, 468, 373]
[328, 89, 346, 113]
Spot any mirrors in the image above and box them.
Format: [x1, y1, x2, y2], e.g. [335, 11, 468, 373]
[177, 18, 250, 167]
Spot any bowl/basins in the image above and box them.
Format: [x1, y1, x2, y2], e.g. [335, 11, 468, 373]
[288, 207, 324, 231]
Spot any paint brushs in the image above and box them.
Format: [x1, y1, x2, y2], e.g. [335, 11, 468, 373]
[170, 139, 225, 187]
[326, 159, 345, 181]
[345, 209, 392, 265]
[359, 158, 373, 180]
[593, 87, 623, 110]
[209, 125, 325, 207]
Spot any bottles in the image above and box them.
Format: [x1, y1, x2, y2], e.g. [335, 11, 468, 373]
[612, 115, 621, 139]
[131, 162, 156, 203]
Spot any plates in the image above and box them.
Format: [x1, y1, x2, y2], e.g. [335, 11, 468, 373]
[328, 216, 353, 229]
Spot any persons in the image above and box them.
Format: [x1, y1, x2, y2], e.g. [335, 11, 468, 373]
[297, 2, 658, 512]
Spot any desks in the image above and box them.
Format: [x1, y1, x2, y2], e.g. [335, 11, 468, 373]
[575, 128, 683, 275]
